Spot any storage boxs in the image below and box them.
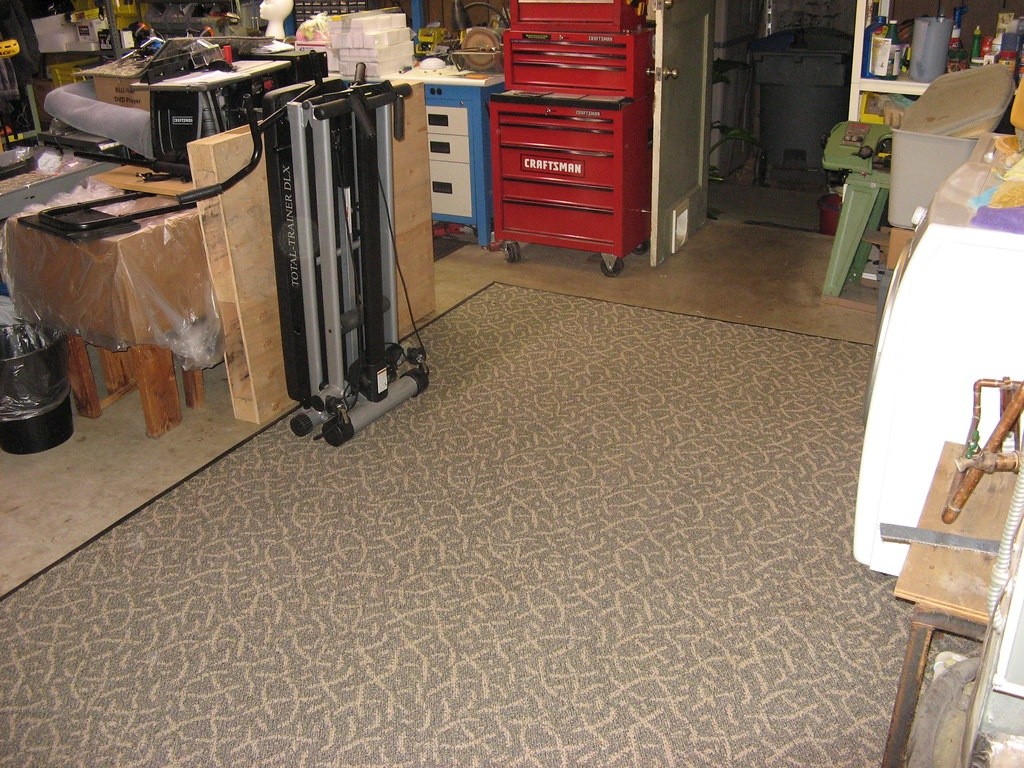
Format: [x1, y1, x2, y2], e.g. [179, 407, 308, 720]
[887, 123, 979, 230]
[46, 57, 100, 89]
[296, 14, 414, 78]
[93, 75, 150, 110]
[885, 225, 915, 271]
[6, 197, 210, 343]
[30, 0, 138, 53]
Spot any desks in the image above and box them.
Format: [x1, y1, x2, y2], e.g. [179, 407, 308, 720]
[880, 440, 1024, 768]
[820, 122, 892, 297]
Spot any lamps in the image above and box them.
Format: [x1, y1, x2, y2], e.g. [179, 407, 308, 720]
[450, 0, 510, 33]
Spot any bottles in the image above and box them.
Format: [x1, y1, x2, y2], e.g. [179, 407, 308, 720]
[862, 16, 890, 78]
[876, 20, 901, 82]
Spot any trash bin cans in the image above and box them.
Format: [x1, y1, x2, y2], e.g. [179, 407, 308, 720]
[750, 26, 854, 183]
[0, 324, 74, 455]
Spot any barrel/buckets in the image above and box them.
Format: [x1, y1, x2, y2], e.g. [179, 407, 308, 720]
[888, 125, 978, 230]
[818, 194, 843, 237]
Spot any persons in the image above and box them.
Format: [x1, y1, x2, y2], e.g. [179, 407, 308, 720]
[257, 0, 295, 40]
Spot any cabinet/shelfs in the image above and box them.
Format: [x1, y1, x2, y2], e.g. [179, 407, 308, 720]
[848, 0, 1018, 122]
[378, 65, 504, 249]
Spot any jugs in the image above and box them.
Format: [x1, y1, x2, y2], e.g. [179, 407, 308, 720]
[908, 17, 951, 83]
